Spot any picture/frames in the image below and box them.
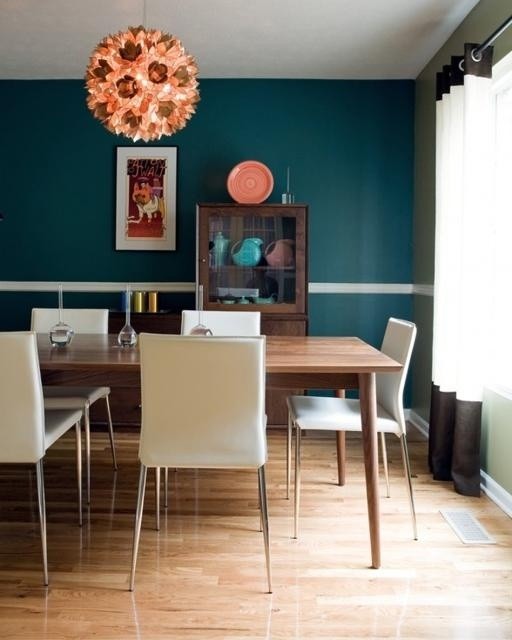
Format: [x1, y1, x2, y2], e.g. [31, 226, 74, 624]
[116, 147, 177, 250]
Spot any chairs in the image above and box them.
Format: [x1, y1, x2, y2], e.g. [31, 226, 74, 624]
[0, 330, 82, 586]
[164, 308, 261, 508]
[30, 307, 118, 504]
[286, 317, 418, 540]
[128, 332, 272, 594]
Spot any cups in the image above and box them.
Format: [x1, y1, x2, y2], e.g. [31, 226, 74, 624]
[222, 299, 235, 304]
[237, 296, 249, 304]
[212, 232, 230, 264]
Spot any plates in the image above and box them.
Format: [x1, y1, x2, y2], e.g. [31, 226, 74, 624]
[226, 160, 275, 204]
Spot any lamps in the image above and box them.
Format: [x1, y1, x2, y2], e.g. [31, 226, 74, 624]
[80, 0, 202, 144]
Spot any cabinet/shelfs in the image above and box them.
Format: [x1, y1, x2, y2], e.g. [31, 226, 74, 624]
[198, 201, 308, 434]
[40, 312, 181, 432]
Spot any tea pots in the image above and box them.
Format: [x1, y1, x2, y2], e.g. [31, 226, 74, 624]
[265, 239, 295, 265]
[253, 293, 279, 304]
[231, 238, 263, 265]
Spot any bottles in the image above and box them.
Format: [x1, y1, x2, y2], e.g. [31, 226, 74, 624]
[49, 282, 74, 348]
[121, 289, 158, 313]
[118, 292, 139, 350]
[187, 284, 214, 338]
